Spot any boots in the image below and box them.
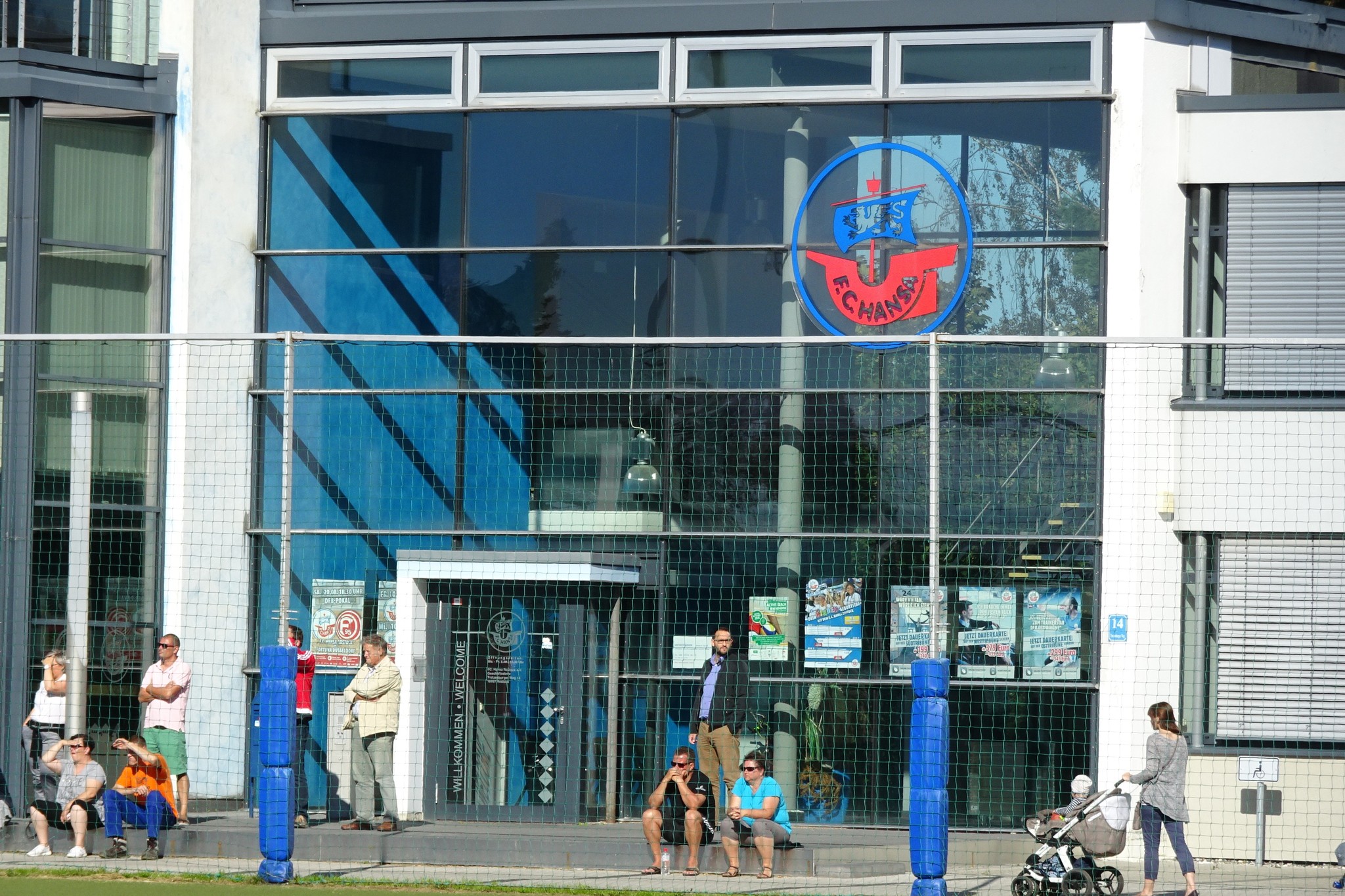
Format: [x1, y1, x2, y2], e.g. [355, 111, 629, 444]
[98, 836, 130, 859]
[141, 837, 159, 860]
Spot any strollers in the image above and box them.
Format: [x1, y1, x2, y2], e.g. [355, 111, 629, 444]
[1010, 776, 1142, 896]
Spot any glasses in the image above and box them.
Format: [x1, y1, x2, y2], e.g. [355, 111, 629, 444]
[742, 766, 759, 772]
[68, 744, 85, 749]
[157, 642, 174, 650]
[670, 761, 691, 768]
[714, 639, 732, 644]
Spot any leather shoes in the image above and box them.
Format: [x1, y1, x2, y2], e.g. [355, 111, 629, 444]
[377, 821, 397, 831]
[341, 820, 374, 830]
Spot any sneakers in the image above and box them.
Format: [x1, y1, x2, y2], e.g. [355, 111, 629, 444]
[293, 815, 307, 828]
[68, 845, 87, 858]
[26, 844, 53, 856]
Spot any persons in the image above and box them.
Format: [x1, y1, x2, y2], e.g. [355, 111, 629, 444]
[641, 746, 715, 875]
[22, 649, 67, 802]
[27, 734, 107, 857]
[749, 598, 782, 641]
[720, 752, 792, 878]
[138, 633, 191, 825]
[806, 578, 862, 616]
[341, 635, 403, 831]
[908, 610, 930, 633]
[1038, 597, 1080, 667]
[99, 735, 179, 859]
[0, 768, 16, 830]
[688, 627, 749, 830]
[958, 601, 1015, 665]
[1122, 702, 1198, 896]
[287, 625, 315, 829]
[1035, 775, 1092, 831]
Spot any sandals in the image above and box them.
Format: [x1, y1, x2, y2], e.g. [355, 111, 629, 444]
[757, 867, 772, 878]
[722, 865, 741, 877]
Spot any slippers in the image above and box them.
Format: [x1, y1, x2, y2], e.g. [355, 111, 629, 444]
[178, 818, 190, 826]
[642, 867, 661, 874]
[683, 867, 699, 876]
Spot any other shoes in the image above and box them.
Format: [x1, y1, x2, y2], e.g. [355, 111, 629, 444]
[1188, 890, 1198, 896]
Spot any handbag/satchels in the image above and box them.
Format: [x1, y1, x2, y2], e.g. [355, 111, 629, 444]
[1132, 802, 1141, 830]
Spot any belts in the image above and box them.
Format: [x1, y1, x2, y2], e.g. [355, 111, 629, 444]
[355, 717, 358, 721]
[699, 718, 709, 724]
[153, 725, 166, 730]
[30, 720, 64, 728]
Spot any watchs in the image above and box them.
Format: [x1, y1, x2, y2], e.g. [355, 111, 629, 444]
[44, 664, 51, 669]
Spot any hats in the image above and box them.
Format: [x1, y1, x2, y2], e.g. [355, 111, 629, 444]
[1071, 774, 1092, 795]
